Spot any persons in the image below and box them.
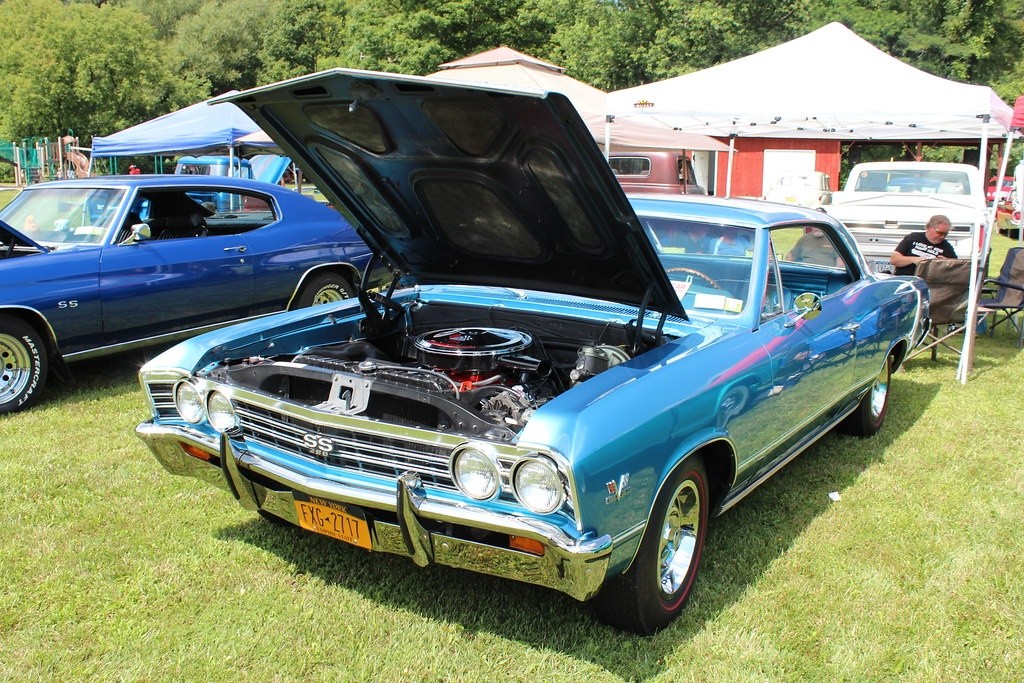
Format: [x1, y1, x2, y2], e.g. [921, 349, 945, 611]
[783, 228, 847, 268]
[660, 224, 717, 256]
[128, 165, 141, 175]
[200, 202, 218, 216]
[708, 227, 755, 256]
[890, 214, 959, 277]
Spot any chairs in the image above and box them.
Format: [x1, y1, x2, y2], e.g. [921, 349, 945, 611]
[914, 253, 989, 362]
[158, 205, 208, 240]
[118, 208, 143, 241]
[936, 178, 964, 193]
[942, 245, 1024, 347]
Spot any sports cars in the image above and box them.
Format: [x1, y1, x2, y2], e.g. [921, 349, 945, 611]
[135, 68, 931, 635]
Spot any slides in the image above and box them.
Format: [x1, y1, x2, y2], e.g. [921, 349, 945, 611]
[66, 152, 90, 178]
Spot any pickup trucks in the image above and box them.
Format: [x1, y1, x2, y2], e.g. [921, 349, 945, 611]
[816, 162, 990, 277]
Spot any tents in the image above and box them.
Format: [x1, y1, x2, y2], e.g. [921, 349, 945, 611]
[611, 21, 1024, 385]
[83, 86, 308, 179]
[238, 41, 737, 200]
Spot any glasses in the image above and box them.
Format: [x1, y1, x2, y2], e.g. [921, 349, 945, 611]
[934, 228, 948, 237]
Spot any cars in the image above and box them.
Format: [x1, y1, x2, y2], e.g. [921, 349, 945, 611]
[986, 177, 1014, 207]
[0, 155, 405, 415]
[603, 152, 705, 195]
